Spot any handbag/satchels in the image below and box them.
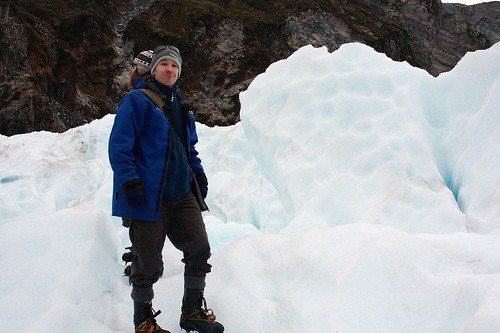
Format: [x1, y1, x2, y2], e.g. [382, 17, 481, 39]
[191, 177, 209, 212]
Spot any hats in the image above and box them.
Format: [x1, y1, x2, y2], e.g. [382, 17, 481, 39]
[150, 46, 183, 78]
[133, 49, 154, 77]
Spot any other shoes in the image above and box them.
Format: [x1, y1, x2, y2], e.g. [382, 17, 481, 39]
[133, 301, 171, 333]
[122, 251, 134, 263]
[124, 265, 164, 276]
[179, 304, 224, 333]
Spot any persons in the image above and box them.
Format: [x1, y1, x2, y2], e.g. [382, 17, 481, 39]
[107, 44, 225, 333]
[122, 50, 164, 276]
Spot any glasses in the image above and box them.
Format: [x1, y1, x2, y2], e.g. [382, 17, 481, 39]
[153, 45, 180, 55]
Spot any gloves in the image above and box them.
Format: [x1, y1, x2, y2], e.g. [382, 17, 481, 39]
[197, 173, 208, 199]
[125, 185, 148, 209]
